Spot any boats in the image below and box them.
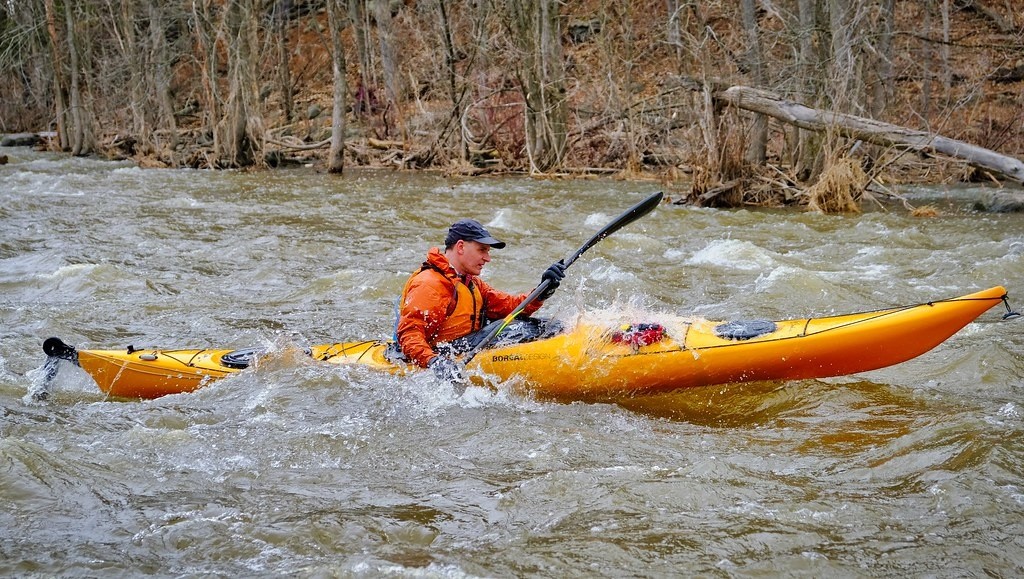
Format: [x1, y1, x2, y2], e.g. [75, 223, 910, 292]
[42, 285, 1009, 401]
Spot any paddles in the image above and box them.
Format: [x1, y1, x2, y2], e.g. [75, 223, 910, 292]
[441, 192, 664, 382]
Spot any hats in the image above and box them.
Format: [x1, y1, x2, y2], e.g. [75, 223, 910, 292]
[445, 219, 506, 250]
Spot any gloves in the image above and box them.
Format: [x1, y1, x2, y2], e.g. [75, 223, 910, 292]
[534, 259, 566, 301]
[428, 358, 461, 381]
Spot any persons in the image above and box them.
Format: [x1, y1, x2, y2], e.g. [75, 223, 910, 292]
[393, 219, 568, 382]
